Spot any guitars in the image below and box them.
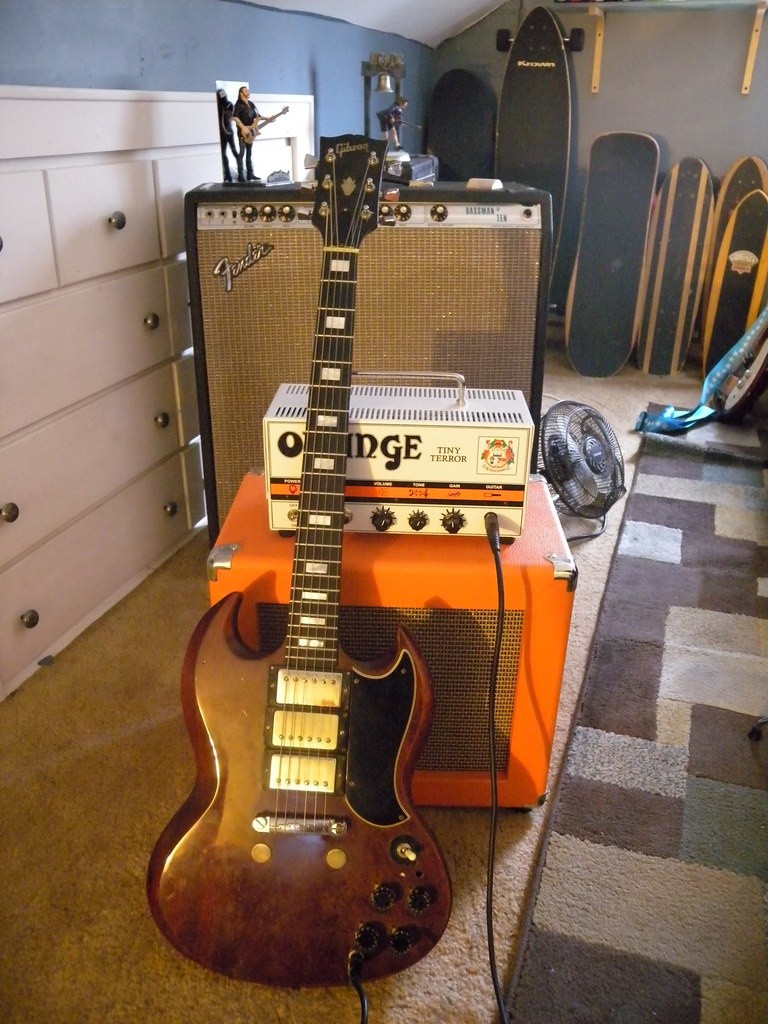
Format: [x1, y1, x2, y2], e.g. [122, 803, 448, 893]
[140, 132, 459, 989]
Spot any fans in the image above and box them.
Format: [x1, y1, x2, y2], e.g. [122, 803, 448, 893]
[536, 400, 626, 541]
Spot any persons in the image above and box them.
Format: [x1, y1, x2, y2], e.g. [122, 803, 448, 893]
[376, 97, 409, 151]
[217, 86, 276, 182]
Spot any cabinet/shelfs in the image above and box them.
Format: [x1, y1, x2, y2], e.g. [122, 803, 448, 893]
[0, 84, 318, 708]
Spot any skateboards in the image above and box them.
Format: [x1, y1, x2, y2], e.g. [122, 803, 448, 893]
[423, 5, 767, 420]
